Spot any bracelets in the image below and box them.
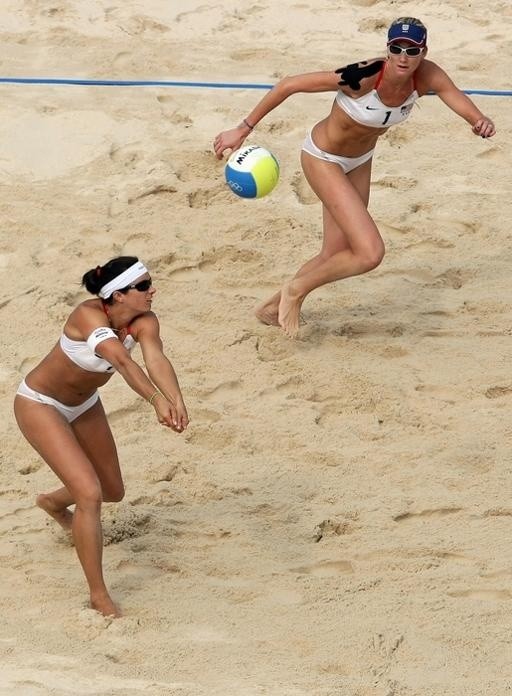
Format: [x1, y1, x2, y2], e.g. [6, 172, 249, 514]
[150, 390, 160, 403]
[243, 117, 254, 131]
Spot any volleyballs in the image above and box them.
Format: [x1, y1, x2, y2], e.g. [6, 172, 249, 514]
[225, 145, 278, 198]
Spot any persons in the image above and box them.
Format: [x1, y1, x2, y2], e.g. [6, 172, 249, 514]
[12, 254, 191, 620]
[211, 13, 495, 339]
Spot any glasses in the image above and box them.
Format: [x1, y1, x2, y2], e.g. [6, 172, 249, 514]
[386, 44, 425, 58]
[121, 277, 153, 292]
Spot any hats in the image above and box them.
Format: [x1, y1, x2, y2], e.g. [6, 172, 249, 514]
[386, 24, 427, 46]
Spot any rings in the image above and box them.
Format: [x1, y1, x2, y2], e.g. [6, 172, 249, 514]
[488, 124, 493, 127]
